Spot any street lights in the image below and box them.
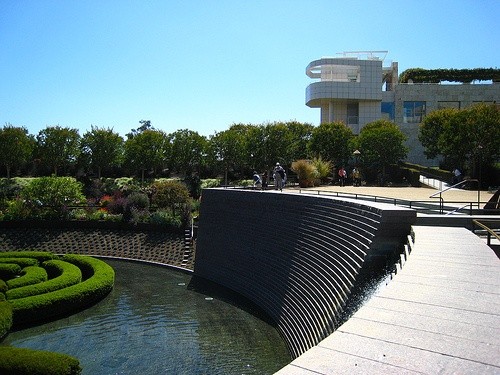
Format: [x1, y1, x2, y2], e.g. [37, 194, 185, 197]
[477, 145, 483, 191]
[353, 150, 361, 186]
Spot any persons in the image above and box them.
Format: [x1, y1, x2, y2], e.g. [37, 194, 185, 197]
[338, 167, 346, 187]
[352, 167, 361, 186]
[282, 170, 287, 188]
[451, 167, 463, 188]
[253, 171, 262, 190]
[261, 170, 269, 190]
[273, 162, 284, 192]
[272, 171, 276, 187]
